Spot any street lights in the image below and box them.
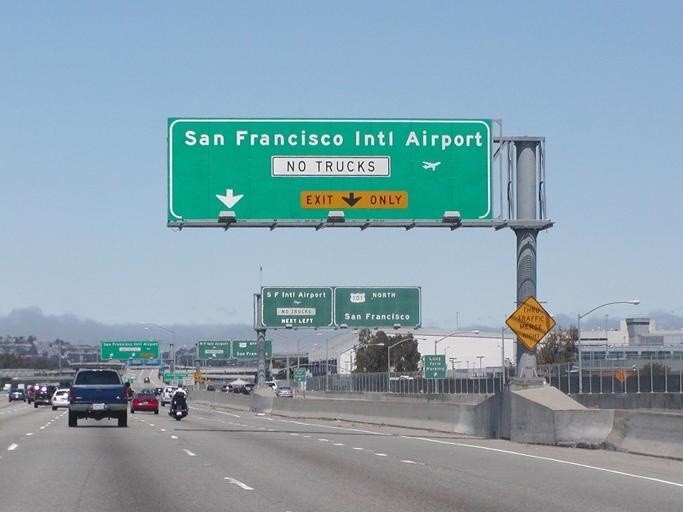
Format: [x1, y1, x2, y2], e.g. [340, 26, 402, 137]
[476, 355, 484, 368]
[139, 322, 176, 373]
[449, 357, 457, 369]
[434, 330, 479, 391]
[348, 343, 384, 390]
[296, 329, 324, 375]
[325, 331, 357, 392]
[577, 299, 640, 394]
[499, 313, 555, 389]
[386, 337, 427, 391]
[443, 345, 450, 356]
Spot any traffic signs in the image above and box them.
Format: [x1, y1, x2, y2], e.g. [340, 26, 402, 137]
[421, 354, 446, 381]
[196, 340, 231, 360]
[334, 287, 421, 330]
[166, 116, 490, 225]
[231, 340, 272, 360]
[259, 286, 332, 328]
[100, 341, 159, 361]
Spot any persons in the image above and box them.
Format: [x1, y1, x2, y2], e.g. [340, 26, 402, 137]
[172, 385, 187, 399]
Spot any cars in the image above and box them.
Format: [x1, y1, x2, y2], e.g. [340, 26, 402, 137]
[1, 368, 189, 427]
[207, 379, 293, 399]
